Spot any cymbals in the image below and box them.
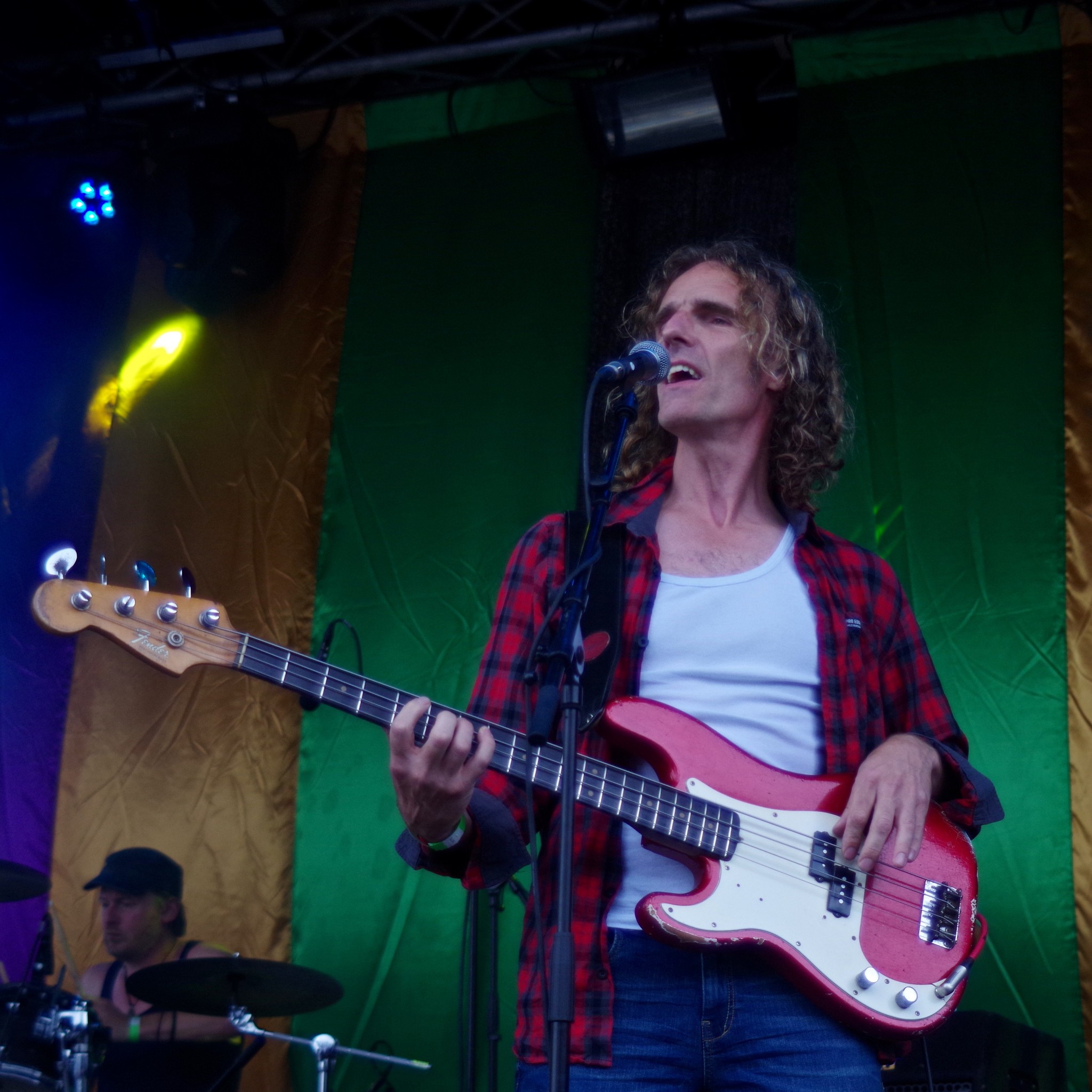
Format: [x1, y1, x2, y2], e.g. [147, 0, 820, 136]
[0, 861, 53, 903]
[123, 957, 346, 1019]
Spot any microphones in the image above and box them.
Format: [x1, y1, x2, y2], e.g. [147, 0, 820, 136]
[593, 340, 672, 386]
[299, 624, 336, 711]
[32, 912, 54, 975]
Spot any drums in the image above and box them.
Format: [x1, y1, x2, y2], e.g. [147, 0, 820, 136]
[1, 981, 113, 1092]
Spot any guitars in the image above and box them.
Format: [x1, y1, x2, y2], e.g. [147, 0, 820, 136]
[31, 552, 989, 1030]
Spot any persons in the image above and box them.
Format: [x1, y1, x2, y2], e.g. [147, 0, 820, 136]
[390, 244, 1004, 1092]
[71, 847, 256, 1092]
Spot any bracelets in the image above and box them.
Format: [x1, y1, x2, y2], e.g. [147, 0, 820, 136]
[127, 1014, 143, 1042]
[418, 812, 469, 854]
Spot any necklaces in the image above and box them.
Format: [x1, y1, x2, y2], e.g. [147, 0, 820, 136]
[122, 939, 178, 1018]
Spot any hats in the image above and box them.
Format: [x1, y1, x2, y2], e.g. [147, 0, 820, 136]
[82, 847, 183, 895]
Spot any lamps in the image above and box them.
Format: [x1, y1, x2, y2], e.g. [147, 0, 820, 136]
[594, 64, 737, 163]
